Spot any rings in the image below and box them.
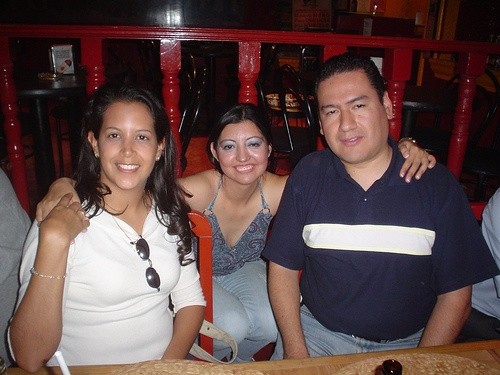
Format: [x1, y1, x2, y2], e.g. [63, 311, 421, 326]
[79, 211, 87, 217]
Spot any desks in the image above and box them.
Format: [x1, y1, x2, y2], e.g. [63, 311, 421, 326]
[0, 341, 500, 375]
[17, 75, 88, 196]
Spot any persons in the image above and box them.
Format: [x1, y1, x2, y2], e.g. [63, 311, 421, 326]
[36, 102, 437, 363]
[453, 186, 500, 343]
[260, 51, 500, 359]
[0, 167, 33, 375]
[8, 80, 207, 374]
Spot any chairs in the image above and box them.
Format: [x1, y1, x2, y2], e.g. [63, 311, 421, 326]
[254, 26, 336, 175]
[185, 210, 214, 363]
[412, 70, 500, 182]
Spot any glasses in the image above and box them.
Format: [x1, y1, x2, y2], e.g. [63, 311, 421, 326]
[136, 234, 162, 291]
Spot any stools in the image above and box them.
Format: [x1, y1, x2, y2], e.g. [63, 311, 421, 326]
[137, 40, 220, 175]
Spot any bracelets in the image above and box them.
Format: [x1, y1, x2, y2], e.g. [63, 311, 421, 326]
[31, 268, 68, 281]
[408, 136, 419, 142]
